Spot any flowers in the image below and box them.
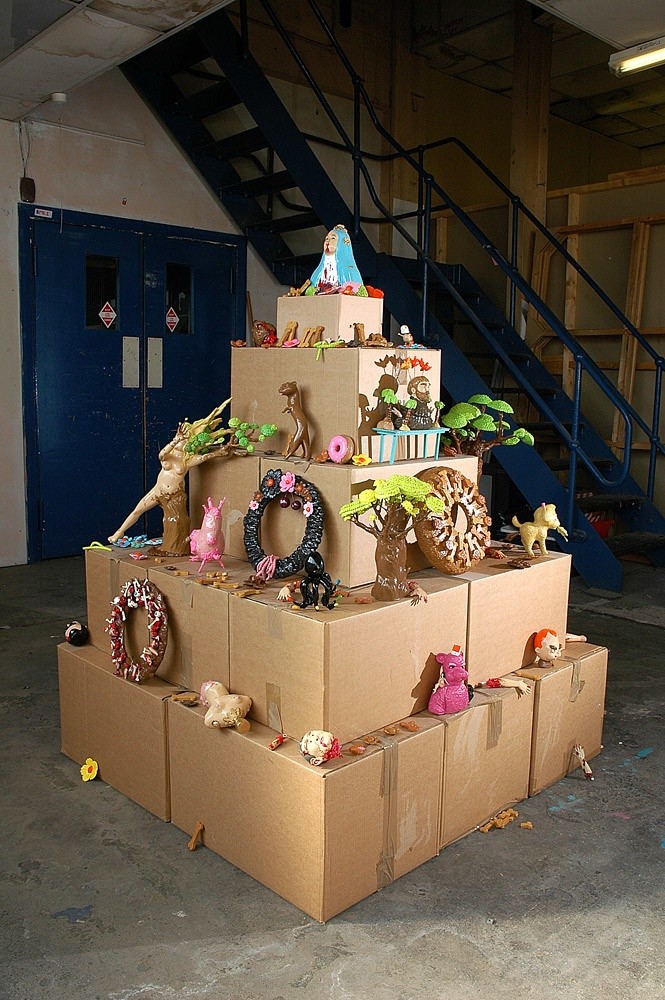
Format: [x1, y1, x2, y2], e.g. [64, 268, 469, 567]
[80, 757, 99, 782]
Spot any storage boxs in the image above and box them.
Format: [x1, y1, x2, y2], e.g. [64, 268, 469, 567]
[57, 292, 610, 922]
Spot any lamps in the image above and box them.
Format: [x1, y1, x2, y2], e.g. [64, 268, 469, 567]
[609, 36, 665, 77]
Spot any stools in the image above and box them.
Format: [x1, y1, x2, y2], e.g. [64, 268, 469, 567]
[372, 427, 449, 465]
